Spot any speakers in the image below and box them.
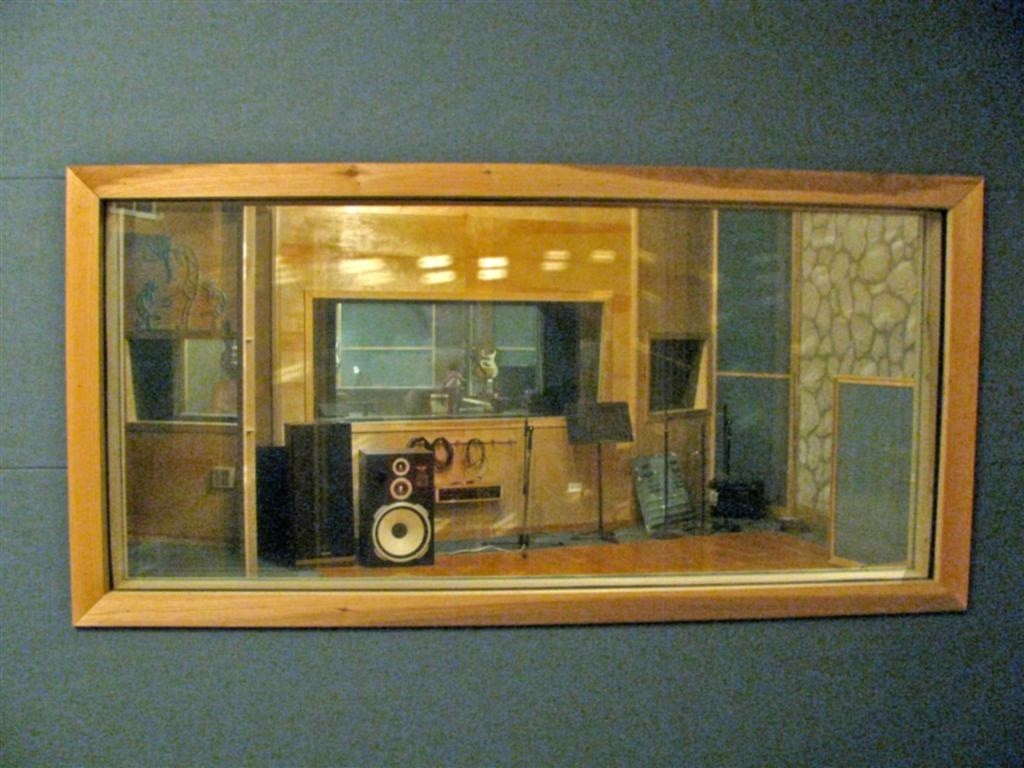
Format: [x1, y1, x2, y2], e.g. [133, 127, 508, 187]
[360, 450, 433, 569]
[283, 422, 354, 559]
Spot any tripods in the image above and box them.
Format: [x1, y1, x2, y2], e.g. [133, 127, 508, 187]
[564, 398, 634, 545]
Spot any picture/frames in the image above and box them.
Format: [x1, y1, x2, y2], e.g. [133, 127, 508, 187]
[59, 161, 984, 614]
